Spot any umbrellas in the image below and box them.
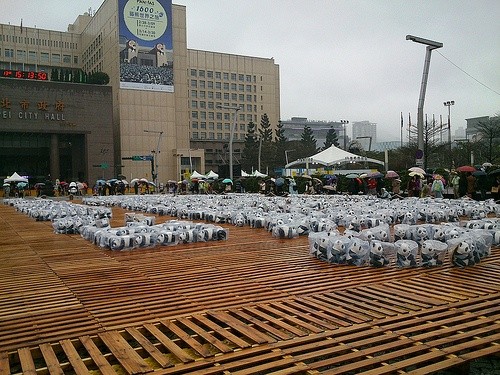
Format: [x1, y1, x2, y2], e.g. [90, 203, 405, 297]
[302, 174, 336, 191]
[488, 169, 500, 175]
[3, 183, 10, 186]
[482, 163, 493, 166]
[472, 171, 487, 176]
[275, 177, 284, 186]
[346, 171, 399, 178]
[456, 166, 476, 171]
[17, 182, 26, 187]
[177, 178, 206, 184]
[131, 178, 156, 187]
[408, 167, 433, 179]
[96, 179, 128, 187]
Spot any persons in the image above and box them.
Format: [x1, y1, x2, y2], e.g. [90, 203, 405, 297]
[409, 174, 444, 198]
[169, 181, 206, 196]
[134, 181, 155, 195]
[5, 185, 24, 199]
[59, 181, 88, 195]
[451, 162, 500, 198]
[92, 181, 126, 196]
[35, 185, 42, 198]
[348, 176, 401, 194]
[258, 176, 337, 196]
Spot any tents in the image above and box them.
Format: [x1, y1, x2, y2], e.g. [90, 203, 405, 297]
[241, 169, 267, 177]
[4, 172, 28, 183]
[190, 170, 219, 179]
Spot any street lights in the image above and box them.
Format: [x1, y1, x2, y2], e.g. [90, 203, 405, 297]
[143, 129, 165, 193]
[285, 149, 296, 165]
[246, 133, 262, 173]
[216, 105, 241, 181]
[405, 34, 444, 170]
[356, 136, 373, 151]
[443, 100, 455, 173]
[340, 120, 349, 151]
